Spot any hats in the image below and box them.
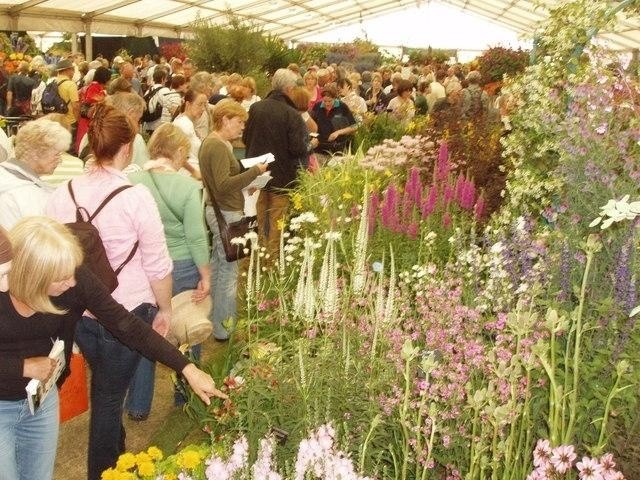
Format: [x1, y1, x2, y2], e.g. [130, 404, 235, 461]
[55, 59, 74, 71]
[162, 290, 214, 350]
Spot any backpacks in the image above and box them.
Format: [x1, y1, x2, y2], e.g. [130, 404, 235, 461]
[63, 178, 139, 297]
[141, 85, 164, 122]
[41, 80, 71, 115]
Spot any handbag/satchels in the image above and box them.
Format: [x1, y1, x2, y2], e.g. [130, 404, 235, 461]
[221, 213, 259, 261]
[80, 101, 90, 118]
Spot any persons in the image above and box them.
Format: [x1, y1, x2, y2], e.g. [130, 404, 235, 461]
[1, 103, 269, 480]
[1, 49, 506, 266]
